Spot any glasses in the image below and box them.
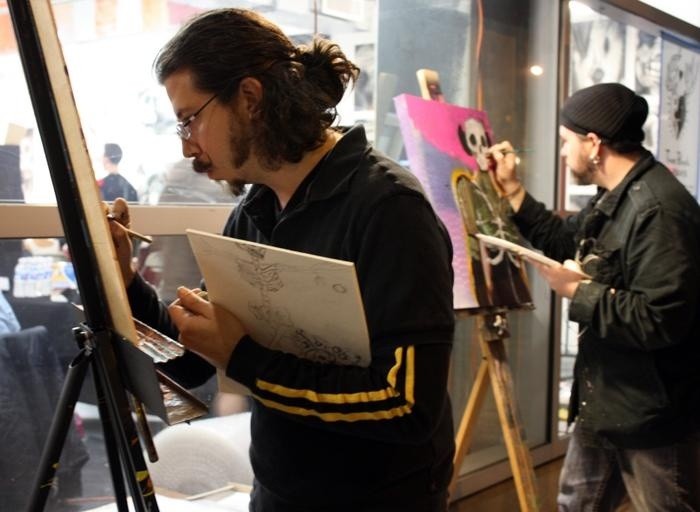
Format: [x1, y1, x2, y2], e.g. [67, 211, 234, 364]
[174, 93, 218, 140]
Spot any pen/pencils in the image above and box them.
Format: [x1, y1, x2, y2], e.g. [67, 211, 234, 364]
[480, 149, 533, 155]
[110, 219, 153, 243]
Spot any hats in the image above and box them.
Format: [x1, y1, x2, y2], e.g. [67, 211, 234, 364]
[560, 83, 648, 142]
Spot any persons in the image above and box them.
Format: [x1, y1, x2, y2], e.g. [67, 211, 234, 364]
[98, 7, 455, 511]
[488, 82, 699, 512]
[144, 141, 245, 304]
[98, 142, 138, 202]
